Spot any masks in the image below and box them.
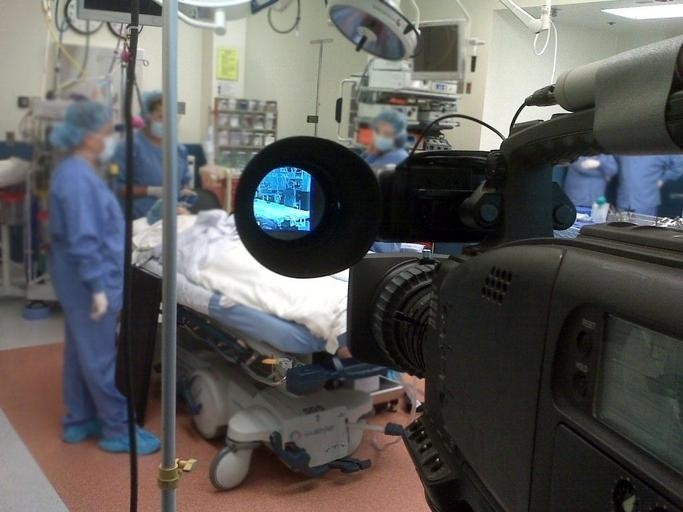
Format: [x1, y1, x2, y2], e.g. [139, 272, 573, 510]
[150, 122, 163, 139]
[99, 132, 120, 163]
[374, 132, 392, 151]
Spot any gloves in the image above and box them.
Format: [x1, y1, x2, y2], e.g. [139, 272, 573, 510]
[91, 291, 109, 321]
[147, 186, 163, 198]
[657, 180, 663, 187]
[581, 160, 601, 168]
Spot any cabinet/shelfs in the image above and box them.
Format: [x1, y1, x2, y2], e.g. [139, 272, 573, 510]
[212, 97, 277, 168]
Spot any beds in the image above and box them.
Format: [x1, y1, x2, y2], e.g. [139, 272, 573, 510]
[125, 209, 422, 491]
[253, 198, 311, 232]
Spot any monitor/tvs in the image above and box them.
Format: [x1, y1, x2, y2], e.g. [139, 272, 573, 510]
[413, 18, 467, 79]
[76, 0, 163, 26]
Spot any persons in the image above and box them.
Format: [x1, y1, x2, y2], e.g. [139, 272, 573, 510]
[48, 101, 160, 455]
[113, 93, 192, 221]
[549, 154, 683, 217]
[342, 107, 410, 255]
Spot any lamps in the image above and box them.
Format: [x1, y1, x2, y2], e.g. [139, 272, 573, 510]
[329, 1, 420, 61]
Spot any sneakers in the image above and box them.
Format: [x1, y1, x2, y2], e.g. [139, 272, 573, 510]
[64, 419, 101, 442]
[99, 426, 160, 455]
